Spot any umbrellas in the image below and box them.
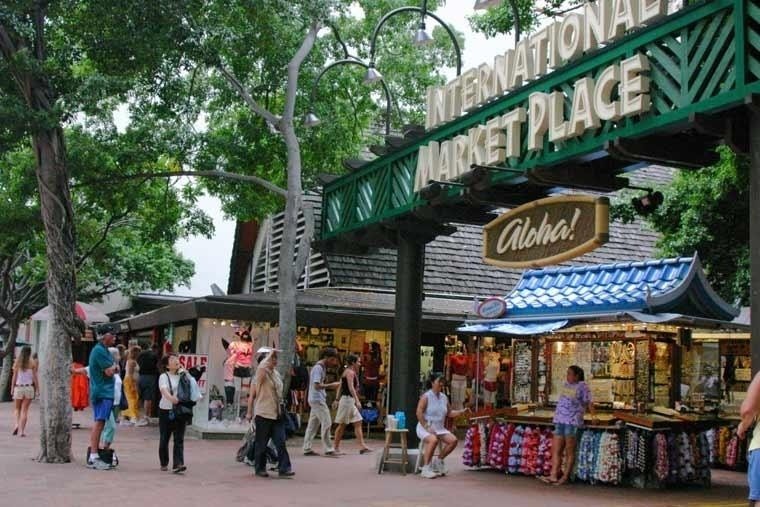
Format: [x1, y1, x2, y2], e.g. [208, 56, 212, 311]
[29, 300, 110, 325]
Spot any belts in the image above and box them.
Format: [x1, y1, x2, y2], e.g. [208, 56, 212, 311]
[15, 384, 33, 387]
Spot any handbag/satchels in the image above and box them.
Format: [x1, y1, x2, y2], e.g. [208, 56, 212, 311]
[87, 447, 118, 467]
[172, 404, 193, 422]
[278, 401, 286, 416]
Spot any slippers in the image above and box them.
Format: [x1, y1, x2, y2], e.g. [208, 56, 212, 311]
[552, 478, 570, 486]
[540, 477, 558, 482]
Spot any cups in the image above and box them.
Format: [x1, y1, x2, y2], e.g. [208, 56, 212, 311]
[386, 414, 394, 429]
[391, 418, 399, 429]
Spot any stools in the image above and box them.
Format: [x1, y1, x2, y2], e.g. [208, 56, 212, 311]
[378, 428, 408, 474]
[415, 438, 444, 474]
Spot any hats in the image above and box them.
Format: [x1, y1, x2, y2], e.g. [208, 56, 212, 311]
[322, 347, 337, 355]
[98, 325, 114, 335]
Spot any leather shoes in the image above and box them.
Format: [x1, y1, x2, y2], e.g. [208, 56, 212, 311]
[279, 470, 295, 476]
[256, 471, 269, 476]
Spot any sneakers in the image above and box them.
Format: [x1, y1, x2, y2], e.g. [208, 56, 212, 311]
[304, 450, 320, 455]
[421, 464, 436, 478]
[160, 466, 167, 470]
[86, 457, 111, 469]
[119, 420, 131, 425]
[12, 427, 18, 434]
[359, 449, 374, 454]
[243, 455, 255, 464]
[433, 458, 448, 476]
[21, 435, 26, 437]
[130, 418, 136, 424]
[172, 464, 187, 472]
[335, 450, 346, 454]
[324, 452, 339, 456]
[135, 420, 148, 426]
[266, 461, 279, 469]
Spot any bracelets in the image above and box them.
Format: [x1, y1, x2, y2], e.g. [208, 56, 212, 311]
[335, 398, 340, 402]
[423, 423, 429, 429]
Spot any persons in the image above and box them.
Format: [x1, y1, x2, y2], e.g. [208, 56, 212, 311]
[70, 347, 123, 450]
[536, 366, 599, 487]
[86, 324, 120, 470]
[415, 372, 472, 478]
[362, 353, 378, 404]
[303, 348, 341, 458]
[736, 370, 760, 507]
[254, 349, 295, 477]
[117, 344, 129, 423]
[332, 355, 375, 454]
[137, 344, 163, 419]
[290, 342, 308, 412]
[158, 351, 197, 473]
[71, 354, 89, 411]
[30, 353, 38, 399]
[446, 352, 469, 410]
[11, 346, 39, 437]
[122, 346, 149, 425]
[482, 353, 500, 410]
[244, 356, 268, 467]
[228, 328, 253, 422]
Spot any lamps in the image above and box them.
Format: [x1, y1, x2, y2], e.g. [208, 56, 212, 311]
[420, 180, 469, 201]
[300, 61, 390, 135]
[414, 1, 518, 53]
[467, 162, 665, 216]
[360, 6, 460, 86]
